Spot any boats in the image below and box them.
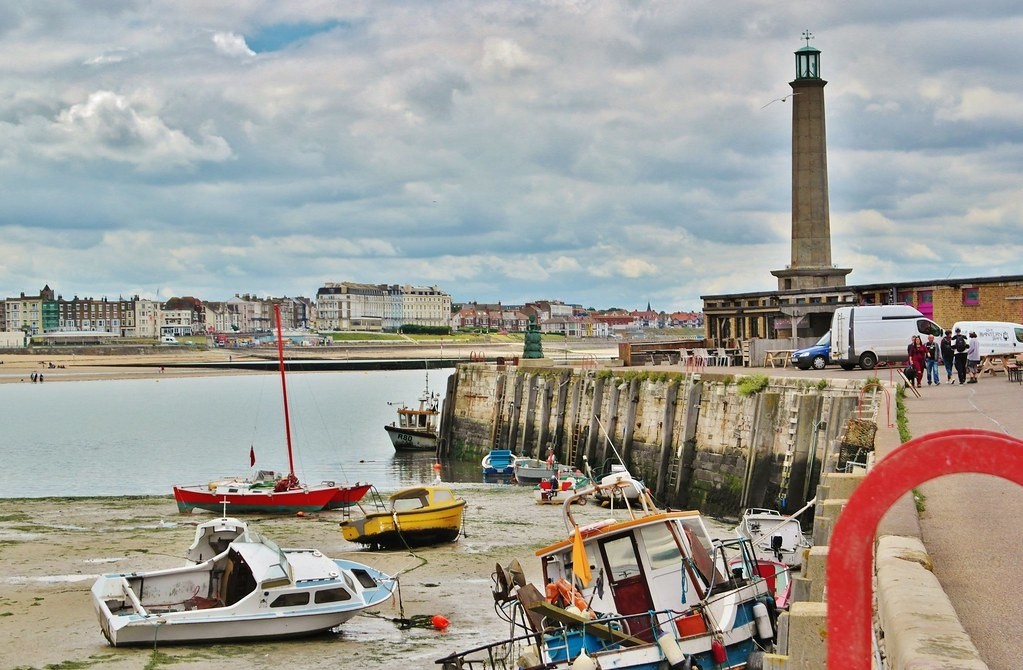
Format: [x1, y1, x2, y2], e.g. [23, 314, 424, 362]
[481, 450, 517, 477]
[513, 435, 570, 485]
[340, 464, 466, 548]
[90, 496, 397, 649]
[723, 556, 791, 613]
[434, 415, 778, 670]
[532, 475, 590, 505]
[384, 371, 440, 452]
[582, 454, 651, 510]
[735, 505, 810, 574]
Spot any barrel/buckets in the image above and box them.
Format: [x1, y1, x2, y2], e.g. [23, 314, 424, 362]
[544, 625, 618, 662]
[674, 613, 707, 637]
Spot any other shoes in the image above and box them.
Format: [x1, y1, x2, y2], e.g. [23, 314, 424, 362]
[928, 382, 932, 386]
[935, 381, 940, 385]
[960, 376, 966, 384]
[947, 378, 955, 385]
[918, 383, 921, 387]
[967, 378, 977, 383]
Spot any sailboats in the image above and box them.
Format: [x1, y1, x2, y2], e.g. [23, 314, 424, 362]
[172, 304, 375, 516]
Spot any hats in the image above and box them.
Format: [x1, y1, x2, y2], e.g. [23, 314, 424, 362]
[956, 328, 960, 332]
[968, 330, 975, 334]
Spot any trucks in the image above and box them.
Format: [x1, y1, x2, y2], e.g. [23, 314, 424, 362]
[828, 305, 946, 371]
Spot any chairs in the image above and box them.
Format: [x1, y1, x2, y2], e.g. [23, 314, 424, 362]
[679, 348, 730, 368]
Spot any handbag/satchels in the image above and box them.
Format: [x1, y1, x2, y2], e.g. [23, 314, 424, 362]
[904, 365, 918, 378]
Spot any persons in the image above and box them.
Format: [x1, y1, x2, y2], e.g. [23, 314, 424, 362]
[22, 360, 65, 384]
[158, 366, 166, 373]
[907, 327, 981, 388]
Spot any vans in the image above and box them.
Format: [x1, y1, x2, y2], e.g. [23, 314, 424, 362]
[951, 321, 1023, 368]
[162, 337, 179, 345]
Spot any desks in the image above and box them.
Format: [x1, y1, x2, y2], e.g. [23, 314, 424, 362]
[977, 352, 1021, 378]
[764, 349, 800, 369]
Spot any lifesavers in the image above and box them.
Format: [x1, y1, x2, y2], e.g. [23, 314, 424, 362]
[569, 518, 617, 537]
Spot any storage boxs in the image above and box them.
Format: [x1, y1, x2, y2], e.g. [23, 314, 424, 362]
[544, 624, 620, 663]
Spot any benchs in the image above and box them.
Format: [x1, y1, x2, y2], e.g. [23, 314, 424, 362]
[763, 356, 795, 361]
[977, 362, 1016, 371]
[1007, 364, 1018, 382]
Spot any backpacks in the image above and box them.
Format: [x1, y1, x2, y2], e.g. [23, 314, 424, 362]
[956, 335, 966, 352]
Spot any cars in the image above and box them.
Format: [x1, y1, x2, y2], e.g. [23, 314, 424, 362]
[790, 330, 839, 370]
